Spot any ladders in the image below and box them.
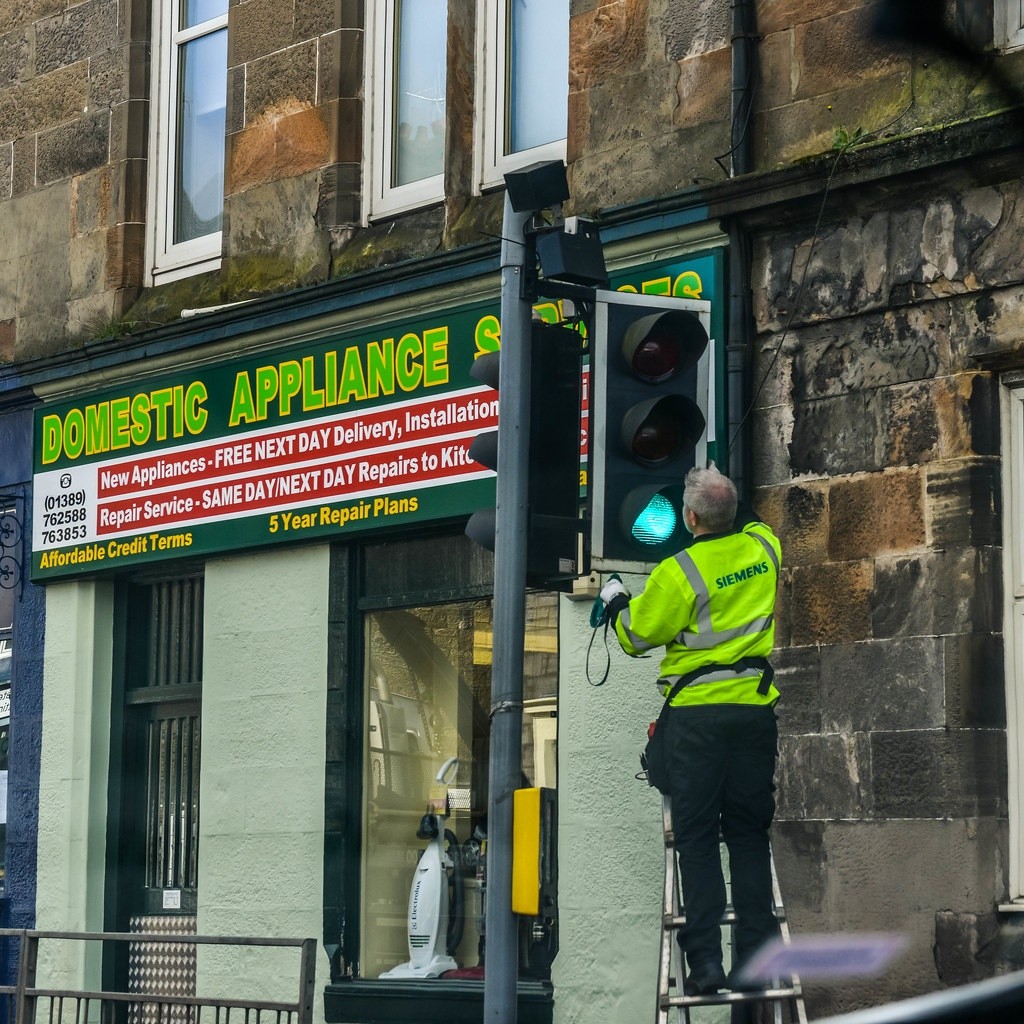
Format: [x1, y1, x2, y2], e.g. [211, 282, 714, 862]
[656, 797, 809, 1024]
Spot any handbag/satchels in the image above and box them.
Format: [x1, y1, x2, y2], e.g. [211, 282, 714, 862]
[640, 657, 774, 795]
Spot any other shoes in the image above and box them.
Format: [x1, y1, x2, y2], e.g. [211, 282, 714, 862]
[684, 963, 727, 995]
[727, 972, 763, 991]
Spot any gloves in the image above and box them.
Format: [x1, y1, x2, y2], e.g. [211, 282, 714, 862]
[598, 579, 629, 604]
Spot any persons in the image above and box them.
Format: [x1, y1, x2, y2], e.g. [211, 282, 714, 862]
[599, 467, 782, 993]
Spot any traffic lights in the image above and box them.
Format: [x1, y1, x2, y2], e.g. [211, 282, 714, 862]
[584, 288, 710, 576]
[466, 320, 582, 596]
